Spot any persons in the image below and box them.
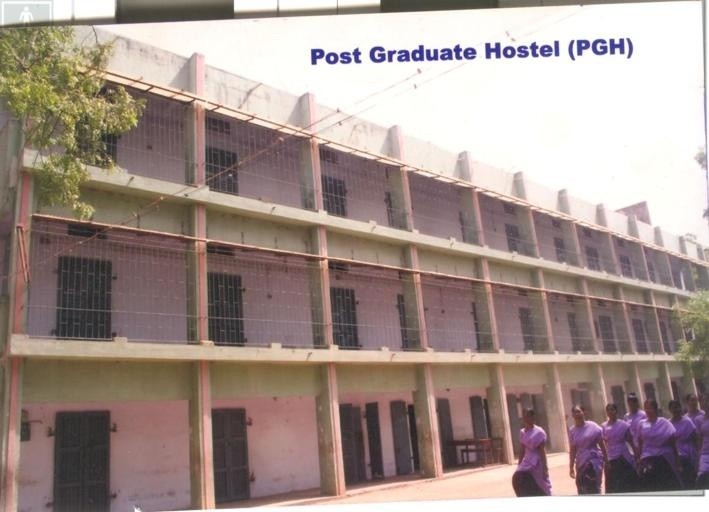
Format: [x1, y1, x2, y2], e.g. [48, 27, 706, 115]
[683, 392, 705, 428]
[567, 404, 611, 496]
[622, 391, 649, 455]
[694, 391, 709, 489]
[597, 402, 643, 494]
[635, 398, 685, 491]
[511, 406, 552, 495]
[665, 397, 702, 488]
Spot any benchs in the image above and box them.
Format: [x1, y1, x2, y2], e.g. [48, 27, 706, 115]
[459, 437, 503, 464]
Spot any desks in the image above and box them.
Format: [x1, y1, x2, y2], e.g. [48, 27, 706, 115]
[442, 438, 493, 465]
